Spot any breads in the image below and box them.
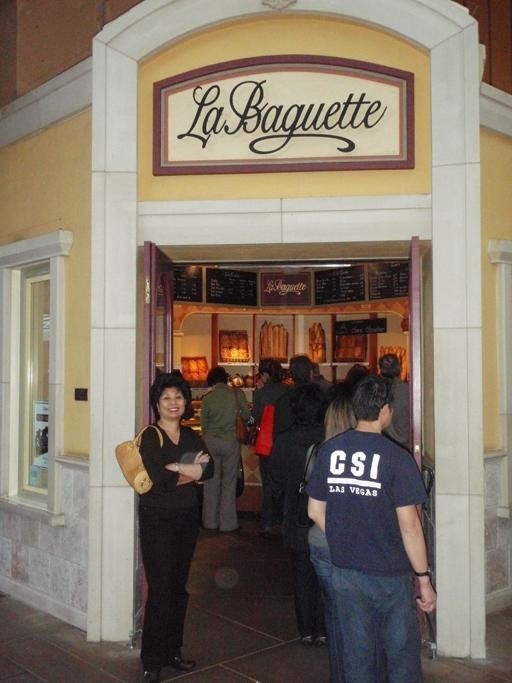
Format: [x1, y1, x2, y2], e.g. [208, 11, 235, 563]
[182, 319, 408, 387]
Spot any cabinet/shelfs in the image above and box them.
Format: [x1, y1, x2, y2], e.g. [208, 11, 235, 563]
[212, 314, 377, 410]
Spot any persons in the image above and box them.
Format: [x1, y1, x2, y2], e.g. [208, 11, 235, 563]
[303, 375, 437, 683]
[199, 352, 410, 537]
[137, 371, 215, 682]
[271, 383, 327, 648]
[304, 392, 358, 683]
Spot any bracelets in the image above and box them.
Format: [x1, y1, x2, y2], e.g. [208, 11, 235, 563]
[174, 460, 179, 474]
[414, 564, 432, 577]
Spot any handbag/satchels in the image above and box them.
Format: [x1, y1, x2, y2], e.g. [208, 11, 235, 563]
[116, 441, 153, 494]
[236, 404, 274, 457]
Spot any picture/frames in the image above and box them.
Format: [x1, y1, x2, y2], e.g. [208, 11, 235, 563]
[31, 400, 50, 472]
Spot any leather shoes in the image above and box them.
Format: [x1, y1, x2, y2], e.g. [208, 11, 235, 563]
[163, 656, 195, 670]
[301, 634, 327, 647]
[143, 670, 160, 683]
